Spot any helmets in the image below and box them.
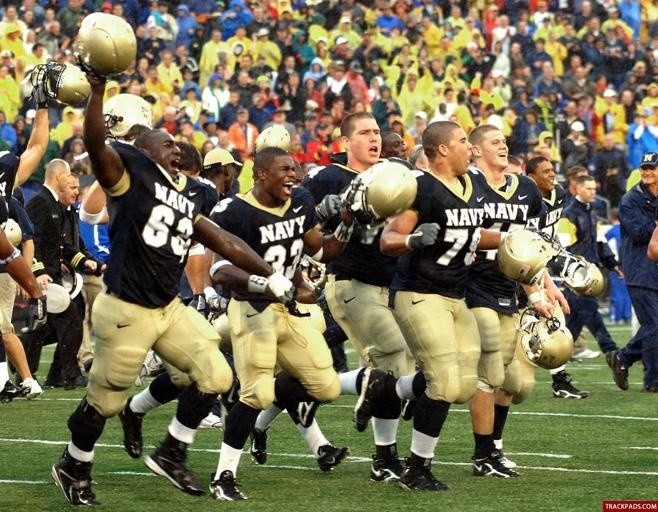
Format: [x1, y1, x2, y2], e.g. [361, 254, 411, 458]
[519, 300, 574, 369]
[563, 255, 605, 297]
[256, 126, 291, 152]
[343, 160, 418, 225]
[103, 93, 153, 140]
[30, 11, 137, 106]
[495, 231, 553, 286]
[3, 218, 22, 246]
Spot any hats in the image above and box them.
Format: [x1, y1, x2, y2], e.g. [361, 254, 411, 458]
[42, 283, 71, 314]
[62, 261, 83, 299]
[202, 148, 244, 170]
[639, 152, 658, 167]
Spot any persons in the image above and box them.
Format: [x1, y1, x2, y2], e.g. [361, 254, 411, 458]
[0, 0, 658, 503]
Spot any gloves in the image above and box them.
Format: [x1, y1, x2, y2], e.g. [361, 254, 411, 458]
[188, 300, 207, 318]
[316, 194, 343, 222]
[405, 222, 440, 251]
[26, 296, 48, 332]
[266, 271, 296, 304]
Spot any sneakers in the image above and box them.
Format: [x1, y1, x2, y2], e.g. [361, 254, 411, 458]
[50, 451, 102, 506]
[0, 361, 95, 401]
[472, 438, 520, 478]
[118, 378, 270, 501]
[135, 350, 161, 387]
[317, 445, 348, 471]
[552, 348, 629, 398]
[296, 402, 319, 427]
[352, 367, 447, 491]
